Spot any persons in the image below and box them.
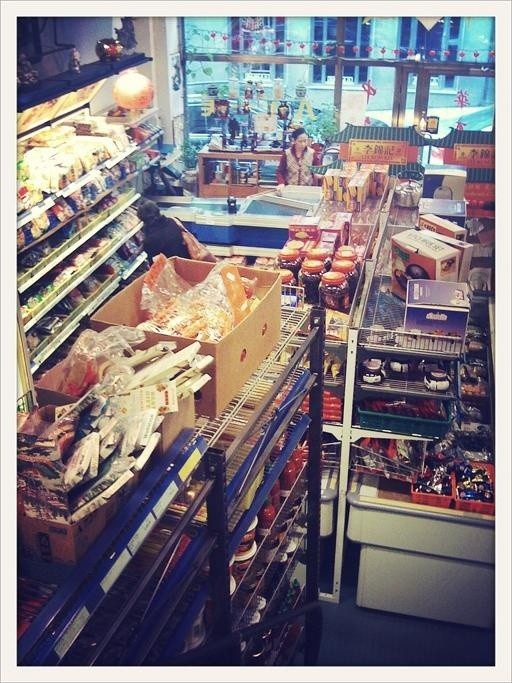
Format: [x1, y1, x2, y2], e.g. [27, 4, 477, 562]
[137, 200, 192, 267]
[276, 128, 321, 197]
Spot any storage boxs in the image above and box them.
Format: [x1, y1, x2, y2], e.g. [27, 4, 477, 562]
[33, 355, 196, 456]
[418, 213, 467, 242]
[411, 467, 454, 508]
[402, 278, 470, 355]
[422, 169, 467, 200]
[453, 463, 495, 514]
[17, 492, 119, 567]
[418, 197, 466, 228]
[90, 257, 282, 419]
[391, 229, 473, 301]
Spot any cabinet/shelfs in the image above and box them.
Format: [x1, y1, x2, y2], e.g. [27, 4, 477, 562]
[196, 141, 283, 199]
[17, 255, 321, 665]
[347, 169, 495, 631]
[247, 253, 363, 603]
[17, 48, 182, 413]
[152, 177, 386, 258]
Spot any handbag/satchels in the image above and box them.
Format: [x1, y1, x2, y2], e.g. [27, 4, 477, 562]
[168, 216, 217, 263]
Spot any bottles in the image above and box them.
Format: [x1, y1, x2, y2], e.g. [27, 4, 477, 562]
[269, 482, 282, 512]
[240, 580, 257, 608]
[263, 539, 278, 565]
[292, 449, 303, 476]
[258, 500, 276, 539]
[329, 260, 359, 303]
[277, 268, 296, 296]
[318, 272, 349, 316]
[280, 462, 295, 503]
[303, 248, 332, 271]
[298, 260, 325, 305]
[277, 251, 301, 279]
[335, 246, 361, 270]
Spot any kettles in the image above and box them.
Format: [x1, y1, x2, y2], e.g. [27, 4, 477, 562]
[393, 169, 424, 208]
